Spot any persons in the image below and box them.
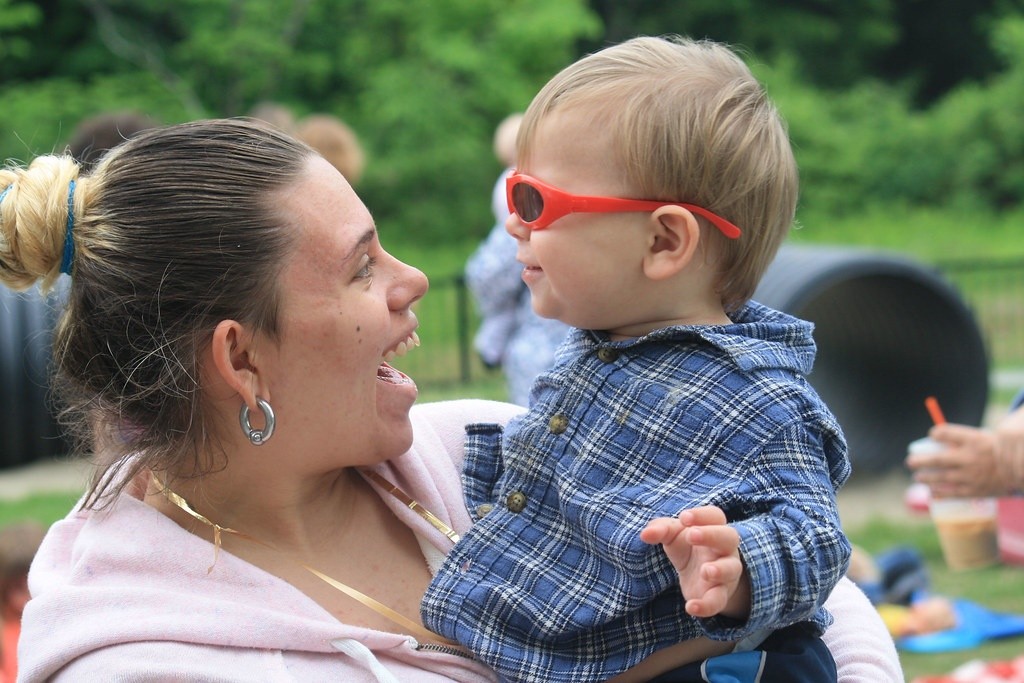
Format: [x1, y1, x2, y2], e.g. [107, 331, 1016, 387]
[254, 105, 365, 186]
[1, 33, 904, 683]
[906, 391, 1023, 499]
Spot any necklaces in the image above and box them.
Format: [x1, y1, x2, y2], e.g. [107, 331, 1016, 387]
[135, 466, 462, 648]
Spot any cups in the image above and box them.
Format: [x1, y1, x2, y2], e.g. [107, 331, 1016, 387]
[929, 496, 1000, 571]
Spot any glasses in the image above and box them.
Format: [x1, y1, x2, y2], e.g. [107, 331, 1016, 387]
[506, 171, 741, 239]
